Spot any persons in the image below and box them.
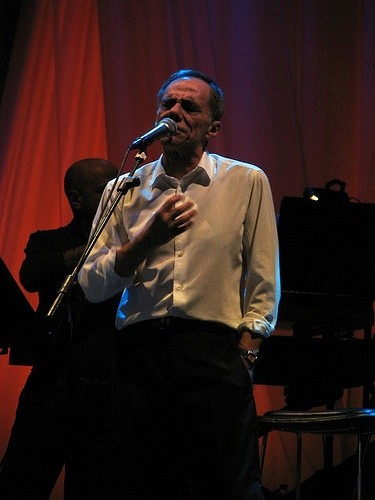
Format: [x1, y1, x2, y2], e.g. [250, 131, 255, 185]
[0, 158, 126, 500]
[77, 69, 281, 500]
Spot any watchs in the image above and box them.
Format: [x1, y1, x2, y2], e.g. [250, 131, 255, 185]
[238, 346, 257, 366]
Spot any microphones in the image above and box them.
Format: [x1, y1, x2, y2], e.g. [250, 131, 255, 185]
[129, 118, 178, 150]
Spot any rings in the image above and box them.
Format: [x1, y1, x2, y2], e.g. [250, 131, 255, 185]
[175, 216, 181, 223]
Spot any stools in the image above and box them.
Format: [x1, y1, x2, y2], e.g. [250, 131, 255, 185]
[260, 407, 375, 500]
